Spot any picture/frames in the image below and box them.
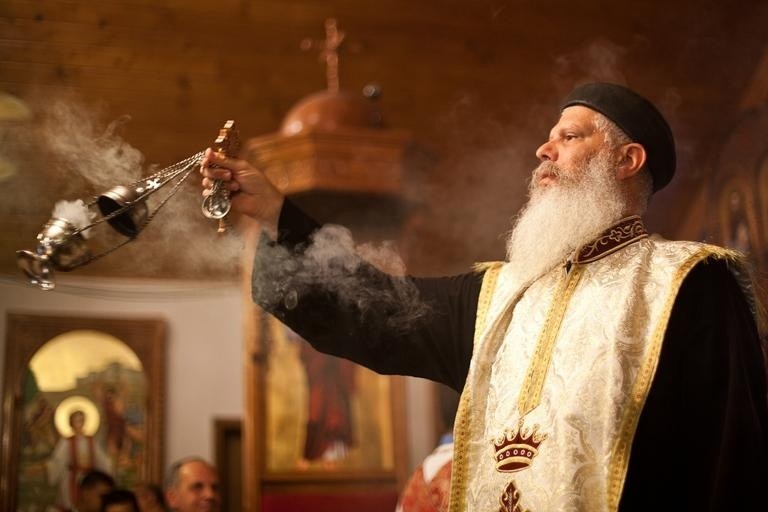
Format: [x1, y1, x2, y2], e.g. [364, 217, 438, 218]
[0, 305, 174, 511]
[245, 272, 409, 484]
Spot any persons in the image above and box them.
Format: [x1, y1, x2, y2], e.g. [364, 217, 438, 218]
[60, 456, 225, 512]
[287, 326, 359, 462]
[199, 82, 766, 512]
[727, 189, 751, 254]
[42, 409, 111, 509]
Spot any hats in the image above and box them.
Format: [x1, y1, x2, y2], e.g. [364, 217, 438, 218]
[559, 82, 676, 195]
